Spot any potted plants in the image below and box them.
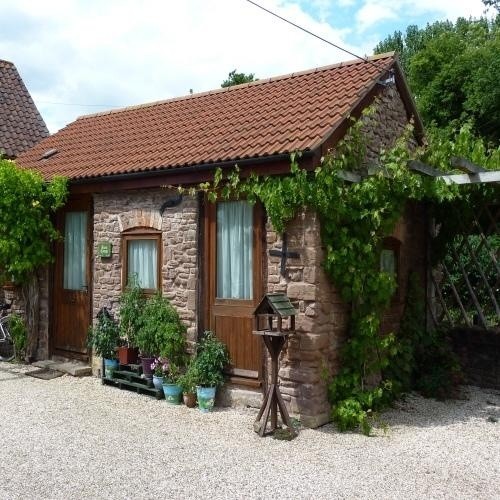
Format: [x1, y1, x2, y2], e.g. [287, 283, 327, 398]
[87, 273, 229, 412]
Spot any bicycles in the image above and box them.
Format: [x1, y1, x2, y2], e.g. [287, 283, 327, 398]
[0, 303, 26, 362]
